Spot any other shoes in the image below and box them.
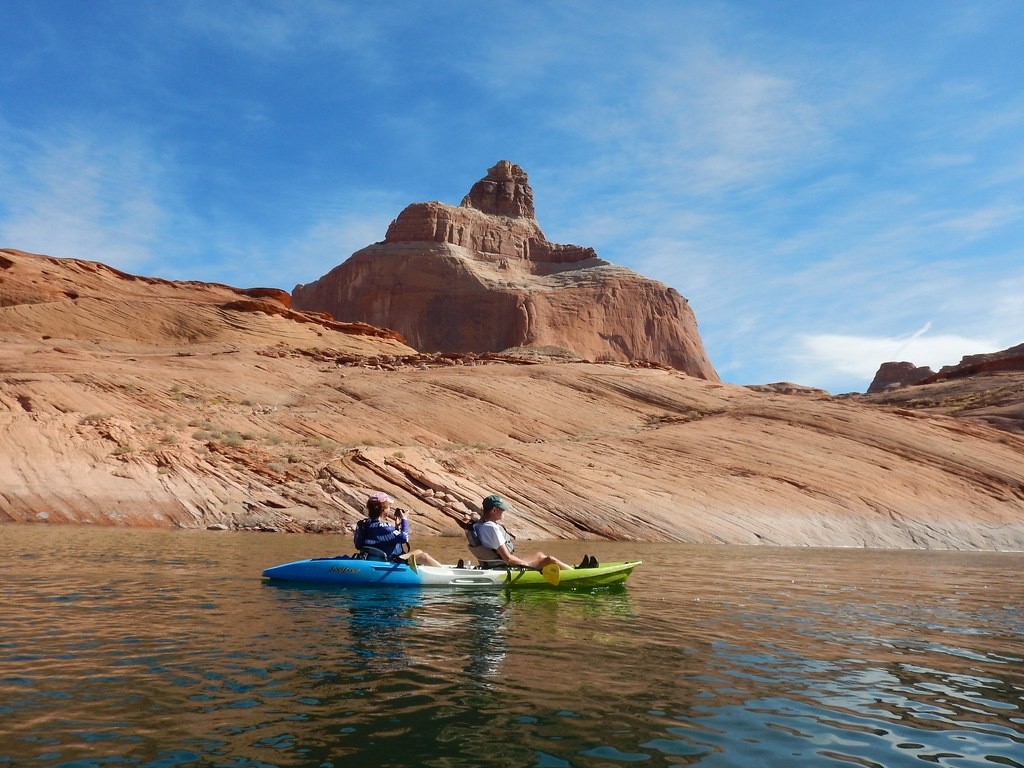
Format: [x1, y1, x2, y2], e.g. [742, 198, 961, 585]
[457, 559, 464, 568]
[589, 556, 599, 569]
[574, 553, 589, 569]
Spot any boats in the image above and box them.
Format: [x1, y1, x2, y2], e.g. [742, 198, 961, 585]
[262, 554, 643, 587]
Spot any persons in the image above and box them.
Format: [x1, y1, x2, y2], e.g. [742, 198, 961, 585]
[467, 495, 599, 572]
[353, 492, 465, 568]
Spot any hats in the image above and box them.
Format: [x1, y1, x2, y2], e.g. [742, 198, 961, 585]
[482, 494, 508, 510]
[370, 491, 394, 504]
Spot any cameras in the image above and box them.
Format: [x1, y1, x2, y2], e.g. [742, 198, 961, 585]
[393, 507, 405, 518]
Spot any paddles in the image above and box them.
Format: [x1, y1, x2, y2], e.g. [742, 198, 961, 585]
[513, 564, 560, 586]
[393, 554, 418, 574]
